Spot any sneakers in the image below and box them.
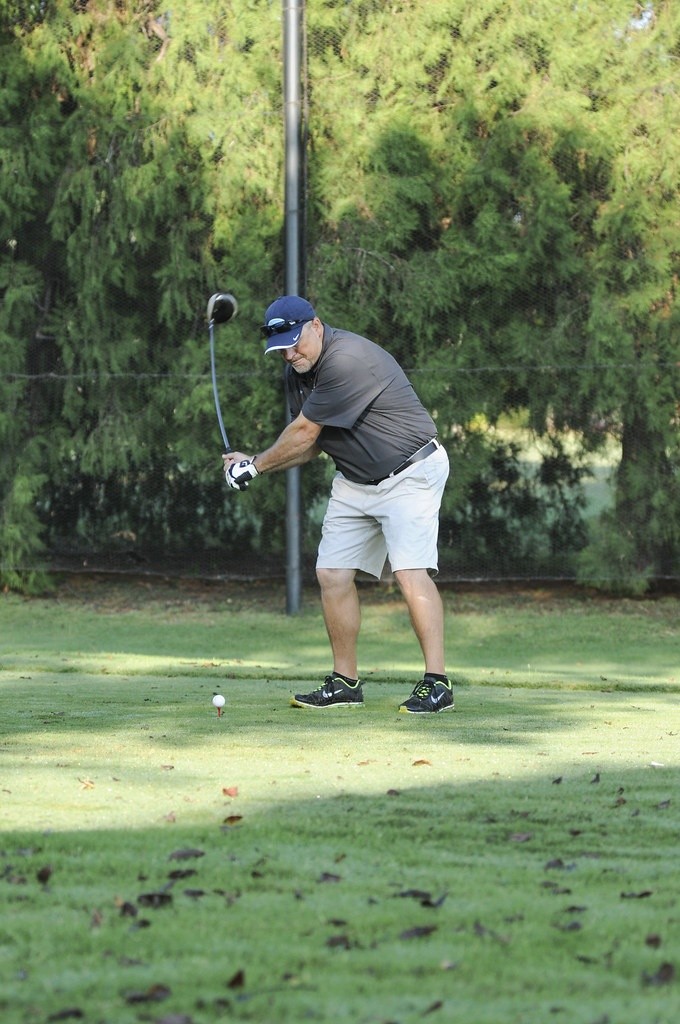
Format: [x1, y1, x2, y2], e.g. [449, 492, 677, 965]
[290, 675, 364, 709]
[398, 677, 455, 714]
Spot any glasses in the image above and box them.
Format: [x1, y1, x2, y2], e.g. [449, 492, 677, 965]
[259, 319, 314, 336]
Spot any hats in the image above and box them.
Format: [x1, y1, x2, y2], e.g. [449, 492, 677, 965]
[264, 295, 315, 355]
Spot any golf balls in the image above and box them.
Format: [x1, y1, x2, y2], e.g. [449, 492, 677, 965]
[213, 694, 226, 707]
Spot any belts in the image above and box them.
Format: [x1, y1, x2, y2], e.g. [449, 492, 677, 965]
[368, 437, 441, 486]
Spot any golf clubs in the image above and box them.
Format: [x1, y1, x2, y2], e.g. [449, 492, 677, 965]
[204, 292, 249, 493]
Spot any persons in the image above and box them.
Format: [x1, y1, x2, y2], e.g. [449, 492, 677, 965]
[221, 296, 454, 714]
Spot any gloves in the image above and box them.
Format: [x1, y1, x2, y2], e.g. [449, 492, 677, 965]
[225, 456, 263, 491]
